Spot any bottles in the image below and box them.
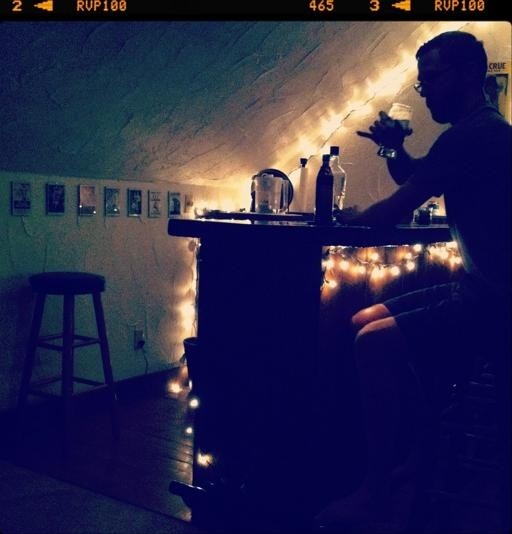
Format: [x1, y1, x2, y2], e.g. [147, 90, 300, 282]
[315, 154, 334, 223]
[413, 207, 432, 227]
[327, 144, 347, 220]
[298, 158, 311, 212]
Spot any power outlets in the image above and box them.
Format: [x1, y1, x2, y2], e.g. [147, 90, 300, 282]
[133, 329, 143, 351]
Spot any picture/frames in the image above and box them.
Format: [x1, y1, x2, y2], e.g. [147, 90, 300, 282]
[484, 68, 512, 126]
[9, 180, 183, 219]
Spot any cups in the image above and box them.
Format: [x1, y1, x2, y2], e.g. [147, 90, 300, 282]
[377, 102, 412, 160]
[252, 171, 289, 214]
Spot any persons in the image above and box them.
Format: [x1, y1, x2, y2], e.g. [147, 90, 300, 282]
[169, 192, 182, 216]
[336, 32, 512, 491]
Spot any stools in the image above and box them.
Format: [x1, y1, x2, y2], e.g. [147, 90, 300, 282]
[15, 271, 117, 419]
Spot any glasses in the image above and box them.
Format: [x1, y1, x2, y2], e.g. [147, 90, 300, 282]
[414, 82, 425, 92]
